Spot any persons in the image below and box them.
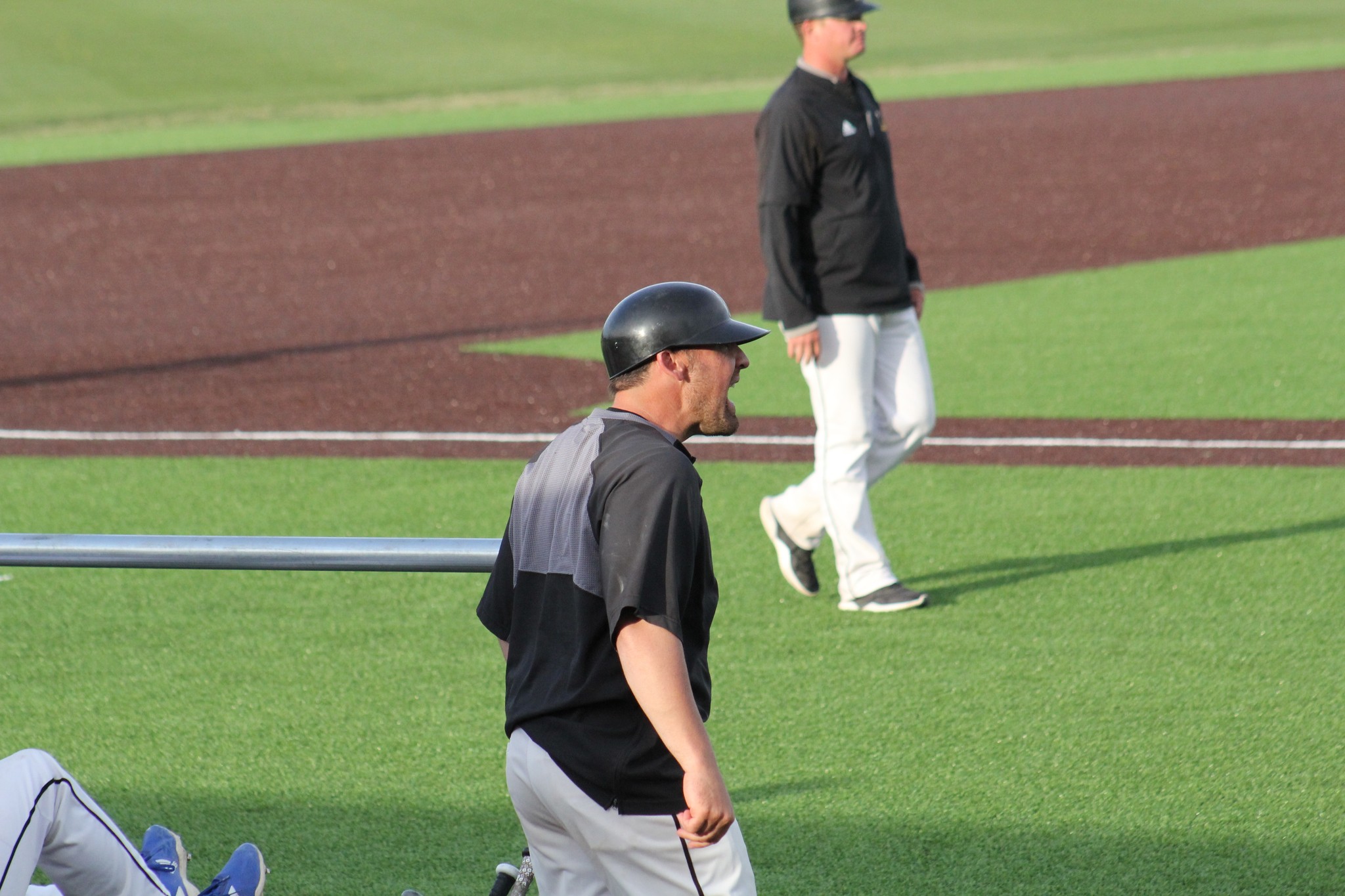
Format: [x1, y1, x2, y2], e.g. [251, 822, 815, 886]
[479, 280, 773, 896]
[750, 1, 937, 614]
[1, 748, 264, 896]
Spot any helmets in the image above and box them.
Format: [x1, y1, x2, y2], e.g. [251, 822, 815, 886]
[601, 281, 771, 378]
[788, 2, 874, 21]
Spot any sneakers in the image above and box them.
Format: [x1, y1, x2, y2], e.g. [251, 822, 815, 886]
[202, 843, 271, 894]
[139, 823, 200, 894]
[758, 496, 821, 598]
[838, 584, 929, 612]
